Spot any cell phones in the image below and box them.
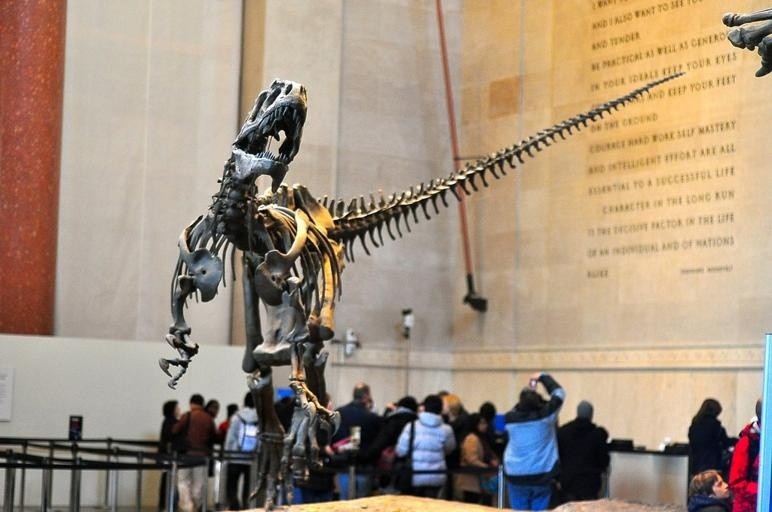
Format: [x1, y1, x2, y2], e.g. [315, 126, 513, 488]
[529, 377, 536, 391]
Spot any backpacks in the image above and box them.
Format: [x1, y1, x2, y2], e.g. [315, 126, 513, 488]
[236, 420, 261, 455]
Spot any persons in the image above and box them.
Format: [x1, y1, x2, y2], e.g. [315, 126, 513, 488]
[728, 395, 763, 511]
[687, 399, 740, 504]
[688, 470, 733, 512]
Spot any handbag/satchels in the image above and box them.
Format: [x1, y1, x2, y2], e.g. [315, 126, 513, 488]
[390, 456, 414, 492]
[171, 412, 193, 454]
